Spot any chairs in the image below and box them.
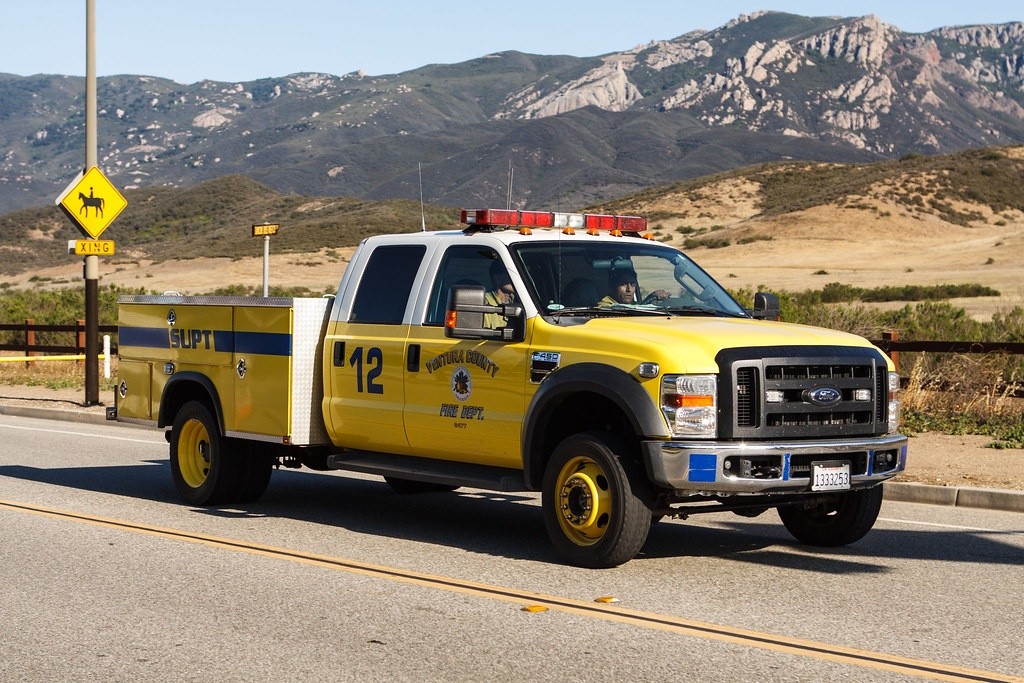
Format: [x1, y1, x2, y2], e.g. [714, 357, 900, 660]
[564, 278, 607, 307]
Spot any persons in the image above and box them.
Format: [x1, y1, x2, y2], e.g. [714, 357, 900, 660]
[596, 268, 670, 306]
[484, 260, 514, 329]
